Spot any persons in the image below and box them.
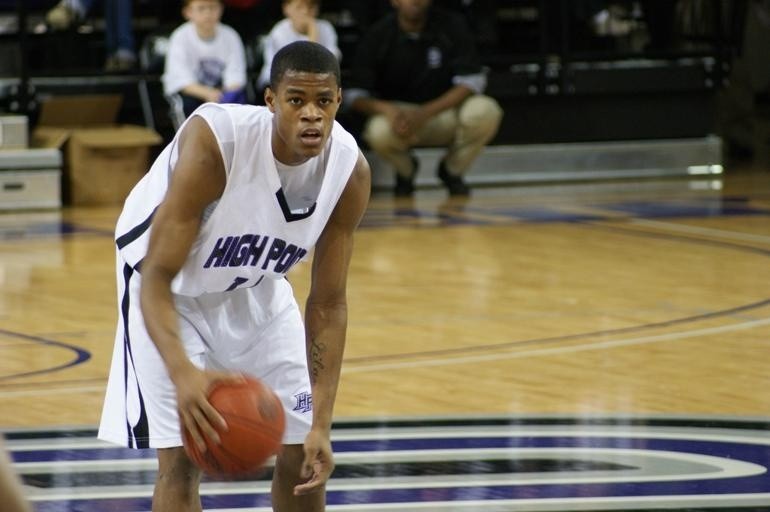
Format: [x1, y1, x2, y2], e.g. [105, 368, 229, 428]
[342, 0, 505, 199]
[255, 0, 347, 95]
[93, 38, 375, 511]
[159, 1, 249, 148]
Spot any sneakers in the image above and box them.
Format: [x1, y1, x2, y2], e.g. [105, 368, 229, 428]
[394, 157, 471, 196]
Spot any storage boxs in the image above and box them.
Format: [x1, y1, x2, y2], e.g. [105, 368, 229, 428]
[32, 92, 166, 204]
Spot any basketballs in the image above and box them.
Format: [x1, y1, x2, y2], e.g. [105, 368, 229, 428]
[182, 373, 285, 479]
